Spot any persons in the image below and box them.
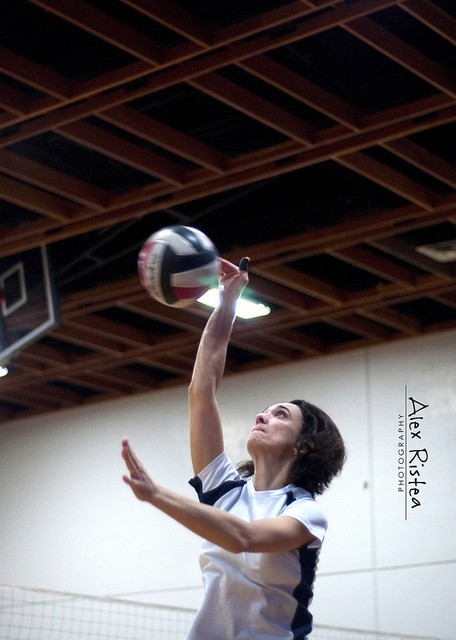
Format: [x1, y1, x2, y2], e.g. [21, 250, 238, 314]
[122, 258, 345, 639]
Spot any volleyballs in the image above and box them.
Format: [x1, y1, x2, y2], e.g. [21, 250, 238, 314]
[139, 225, 219, 308]
